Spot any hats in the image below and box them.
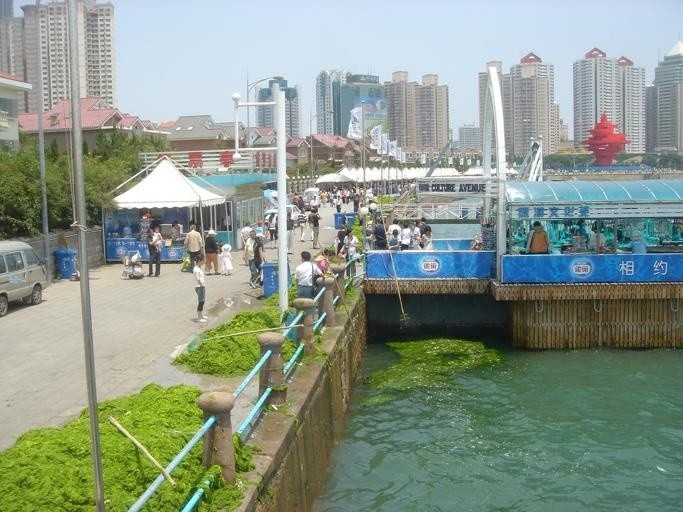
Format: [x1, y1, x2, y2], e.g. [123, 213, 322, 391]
[222, 244, 232, 253]
[208, 229, 218, 235]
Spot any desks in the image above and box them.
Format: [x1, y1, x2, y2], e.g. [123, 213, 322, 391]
[106, 238, 184, 262]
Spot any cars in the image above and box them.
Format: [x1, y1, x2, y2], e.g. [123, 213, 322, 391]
[265, 202, 302, 229]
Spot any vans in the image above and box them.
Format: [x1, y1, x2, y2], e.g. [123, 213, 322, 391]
[302, 186, 321, 209]
[0, 241, 49, 317]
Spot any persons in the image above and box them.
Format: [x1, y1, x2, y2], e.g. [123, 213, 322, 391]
[146, 228, 160, 277]
[217, 244, 234, 276]
[525, 221, 549, 255]
[189, 220, 200, 233]
[184, 224, 204, 274]
[551, 219, 683, 254]
[152, 227, 163, 253]
[240, 187, 437, 297]
[171, 221, 181, 240]
[192, 254, 209, 323]
[205, 229, 222, 275]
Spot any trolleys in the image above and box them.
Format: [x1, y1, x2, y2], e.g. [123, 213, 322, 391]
[122, 249, 143, 280]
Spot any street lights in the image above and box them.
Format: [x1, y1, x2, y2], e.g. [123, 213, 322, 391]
[246, 72, 283, 145]
[361, 206, 368, 251]
[369, 201, 377, 248]
[307, 109, 335, 177]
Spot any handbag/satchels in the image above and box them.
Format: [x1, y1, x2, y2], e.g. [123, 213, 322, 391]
[312, 285, 319, 296]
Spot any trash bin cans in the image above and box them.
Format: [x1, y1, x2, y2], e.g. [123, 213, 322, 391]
[52, 248, 79, 279]
[334, 213, 357, 229]
[260, 262, 292, 298]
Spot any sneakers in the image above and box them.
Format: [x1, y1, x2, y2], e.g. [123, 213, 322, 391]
[197, 315, 209, 323]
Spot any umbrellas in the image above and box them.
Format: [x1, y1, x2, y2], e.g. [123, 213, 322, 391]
[315, 173, 353, 190]
